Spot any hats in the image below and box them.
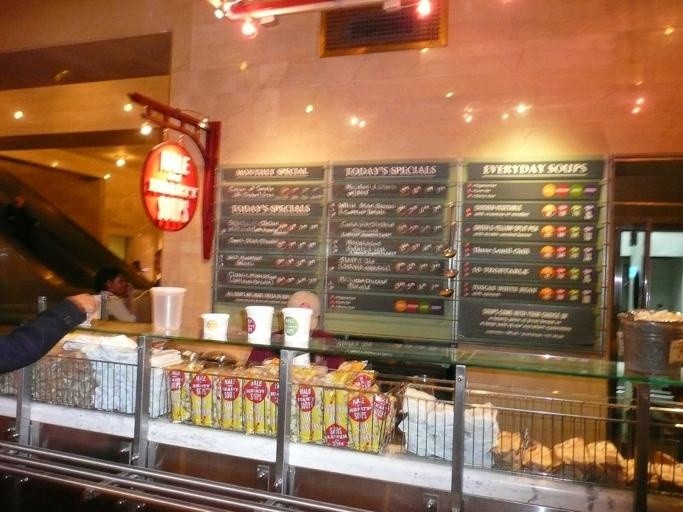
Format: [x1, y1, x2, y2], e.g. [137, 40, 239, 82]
[286, 291, 321, 331]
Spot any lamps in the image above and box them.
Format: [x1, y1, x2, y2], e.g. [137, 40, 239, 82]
[415, 0, 433, 16]
[239, 14, 259, 41]
[112, 154, 128, 168]
[140, 118, 152, 135]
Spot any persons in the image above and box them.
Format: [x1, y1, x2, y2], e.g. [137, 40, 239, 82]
[243, 290, 347, 373]
[92, 267, 141, 322]
[133, 260, 146, 279]
[1, 292, 106, 378]
[0, 196, 40, 240]
[152, 246, 162, 288]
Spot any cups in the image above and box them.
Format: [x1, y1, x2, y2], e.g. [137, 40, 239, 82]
[149, 286, 186, 331]
[200, 313, 230, 341]
[281, 306, 314, 350]
[244, 306, 275, 346]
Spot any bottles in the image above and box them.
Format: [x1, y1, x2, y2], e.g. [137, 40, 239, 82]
[37, 296, 47, 315]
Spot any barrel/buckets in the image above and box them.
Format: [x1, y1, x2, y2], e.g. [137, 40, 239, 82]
[617, 311, 683, 391]
